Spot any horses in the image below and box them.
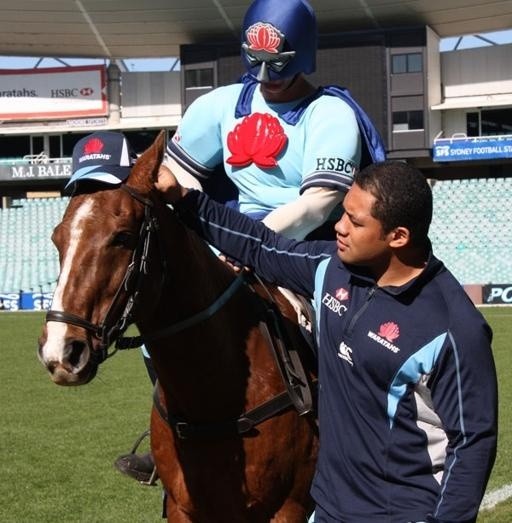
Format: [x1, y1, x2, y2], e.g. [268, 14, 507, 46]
[35, 128, 317, 522]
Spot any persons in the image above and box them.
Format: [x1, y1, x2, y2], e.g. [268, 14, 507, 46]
[154, 162, 496, 523]
[117, 2, 385, 483]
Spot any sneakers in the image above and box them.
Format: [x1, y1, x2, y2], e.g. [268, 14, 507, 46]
[113, 452, 161, 484]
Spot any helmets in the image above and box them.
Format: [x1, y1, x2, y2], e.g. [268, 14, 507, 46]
[241, 0, 319, 85]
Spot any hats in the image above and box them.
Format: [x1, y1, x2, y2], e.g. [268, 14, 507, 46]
[64, 131, 138, 192]
